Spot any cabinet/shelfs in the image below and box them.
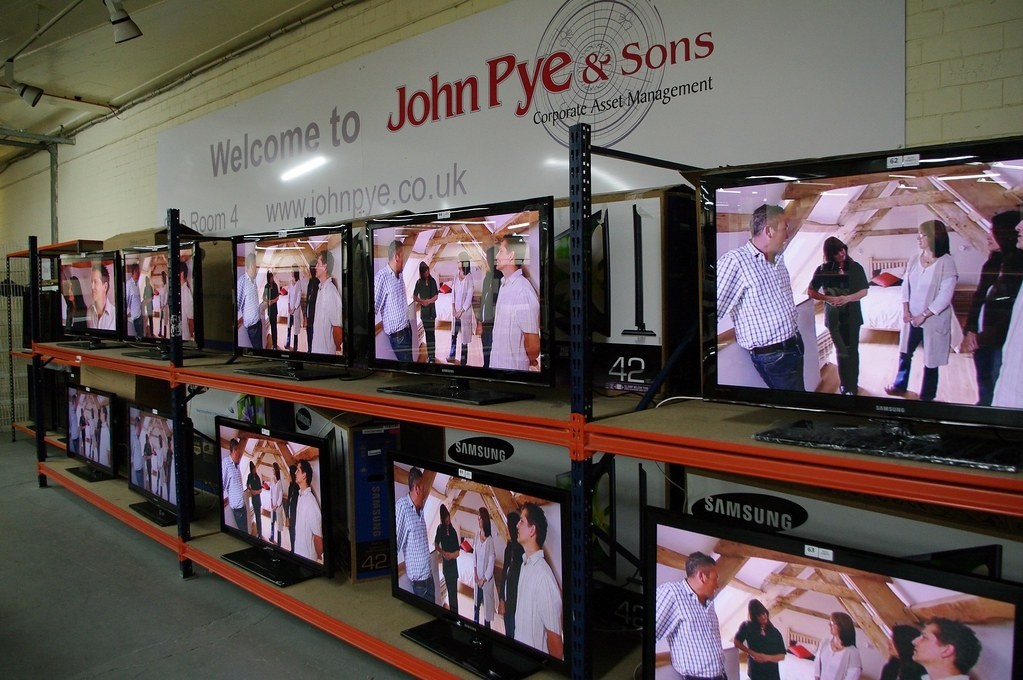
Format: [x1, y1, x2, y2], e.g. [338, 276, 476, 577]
[6, 121, 1023, 680]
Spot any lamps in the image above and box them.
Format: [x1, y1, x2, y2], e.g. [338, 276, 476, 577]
[3, 58, 44, 107]
[102, 0, 143, 45]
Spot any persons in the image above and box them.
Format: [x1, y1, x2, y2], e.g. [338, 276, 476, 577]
[884, 220, 958, 400]
[447, 234, 540, 371]
[61, 264, 116, 330]
[284, 249, 342, 355]
[734, 599, 786, 680]
[237, 253, 279, 351]
[375, 240, 438, 364]
[498, 503, 564, 661]
[808, 237, 869, 395]
[396, 467, 460, 614]
[126, 263, 169, 338]
[472, 507, 495, 629]
[656, 552, 728, 680]
[813, 612, 862, 680]
[222, 438, 324, 565]
[879, 617, 981, 680]
[716, 205, 806, 391]
[179, 262, 195, 340]
[962, 209, 1022, 409]
[69, 394, 111, 468]
[130, 416, 176, 505]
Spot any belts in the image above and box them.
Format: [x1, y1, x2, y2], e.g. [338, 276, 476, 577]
[414, 575, 434, 586]
[753, 332, 801, 355]
[390, 322, 412, 337]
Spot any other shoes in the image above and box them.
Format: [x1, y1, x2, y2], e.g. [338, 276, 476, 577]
[446, 358, 455, 363]
[884, 385, 906, 396]
[838, 385, 858, 395]
[427, 357, 435, 363]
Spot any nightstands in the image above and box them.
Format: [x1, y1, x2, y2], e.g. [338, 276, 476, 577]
[951, 284, 978, 316]
[494, 561, 503, 579]
[472, 292, 482, 308]
[300, 295, 306, 308]
[282, 495, 288, 506]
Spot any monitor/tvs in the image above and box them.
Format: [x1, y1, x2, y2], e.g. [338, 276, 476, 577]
[237, 394, 257, 424]
[554, 209, 611, 337]
[626, 463, 647, 584]
[231, 221, 353, 382]
[556, 458, 618, 580]
[21, 291, 55, 354]
[26, 364, 80, 445]
[56, 250, 126, 351]
[622, 205, 657, 335]
[387, 450, 572, 680]
[701, 134, 1023, 472]
[121, 239, 204, 361]
[126, 402, 196, 527]
[640, 505, 1023, 680]
[364, 196, 554, 405]
[215, 415, 334, 588]
[64, 383, 126, 483]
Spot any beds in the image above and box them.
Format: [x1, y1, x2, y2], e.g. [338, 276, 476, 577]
[858, 256, 965, 355]
[153, 284, 163, 316]
[260, 474, 287, 526]
[456, 525, 499, 614]
[434, 274, 478, 335]
[151, 444, 157, 472]
[778, 627, 823, 680]
[276, 279, 304, 328]
[85, 415, 90, 440]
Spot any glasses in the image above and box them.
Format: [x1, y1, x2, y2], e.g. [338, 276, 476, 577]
[478, 516, 481, 520]
[828, 621, 838, 626]
[916, 233, 927, 239]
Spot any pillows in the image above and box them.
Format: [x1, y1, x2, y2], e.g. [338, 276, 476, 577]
[86, 421, 90, 426]
[282, 285, 289, 290]
[460, 540, 473, 552]
[880, 267, 906, 277]
[443, 281, 452, 287]
[154, 290, 159, 296]
[438, 285, 451, 293]
[153, 450, 157, 455]
[464, 537, 474, 546]
[796, 641, 818, 653]
[262, 483, 270, 490]
[870, 272, 900, 288]
[280, 288, 287, 295]
[788, 645, 815, 658]
